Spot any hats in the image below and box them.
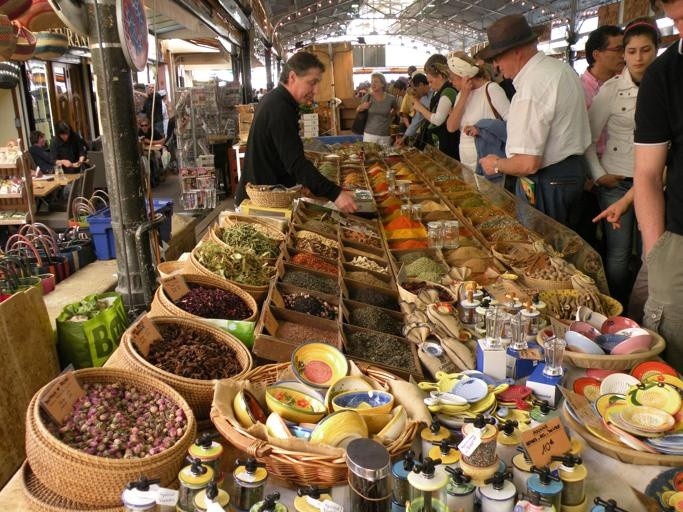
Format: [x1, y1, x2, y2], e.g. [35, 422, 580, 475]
[474, 13, 546, 59]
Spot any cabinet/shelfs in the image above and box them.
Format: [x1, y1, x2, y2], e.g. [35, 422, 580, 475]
[0, 144, 38, 249]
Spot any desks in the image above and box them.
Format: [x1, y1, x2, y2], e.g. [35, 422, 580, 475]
[22, 171, 61, 213]
[42, 169, 87, 207]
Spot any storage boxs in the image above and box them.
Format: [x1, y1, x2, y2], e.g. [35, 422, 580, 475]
[88, 195, 174, 259]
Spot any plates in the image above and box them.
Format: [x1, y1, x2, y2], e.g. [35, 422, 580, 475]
[629, 360, 681, 382]
[647, 434, 683, 454]
[616, 328, 650, 336]
[642, 374, 682, 390]
[565, 331, 606, 354]
[568, 321, 602, 342]
[591, 334, 631, 354]
[583, 415, 633, 449]
[485, 311, 505, 349]
[573, 377, 601, 401]
[601, 418, 660, 453]
[610, 335, 655, 356]
[599, 372, 642, 396]
[629, 384, 682, 415]
[575, 306, 595, 321]
[600, 316, 641, 334]
[587, 312, 609, 334]
[564, 398, 602, 426]
[510, 316, 528, 350]
[609, 405, 663, 436]
[621, 407, 676, 433]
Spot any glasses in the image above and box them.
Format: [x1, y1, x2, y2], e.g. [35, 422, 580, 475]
[606, 46, 621, 52]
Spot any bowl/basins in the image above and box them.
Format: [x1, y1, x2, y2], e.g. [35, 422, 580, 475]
[290, 341, 349, 388]
[287, 425, 311, 438]
[331, 390, 395, 413]
[325, 374, 376, 414]
[300, 421, 316, 429]
[264, 413, 295, 441]
[232, 389, 267, 427]
[371, 404, 410, 446]
[354, 411, 393, 437]
[275, 381, 325, 403]
[310, 409, 369, 450]
[265, 387, 327, 424]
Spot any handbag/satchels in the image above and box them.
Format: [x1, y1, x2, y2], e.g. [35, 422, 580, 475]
[1, 189, 110, 301]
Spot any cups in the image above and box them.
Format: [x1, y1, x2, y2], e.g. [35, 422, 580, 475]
[412, 206, 422, 227]
[542, 337, 566, 377]
[400, 205, 410, 224]
[444, 220, 460, 249]
[428, 222, 443, 250]
[386, 172, 396, 194]
[399, 184, 410, 204]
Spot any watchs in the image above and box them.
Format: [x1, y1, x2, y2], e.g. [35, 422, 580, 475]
[494, 158, 502, 175]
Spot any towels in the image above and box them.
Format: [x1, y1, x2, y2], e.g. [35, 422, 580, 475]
[117, 315, 253, 420]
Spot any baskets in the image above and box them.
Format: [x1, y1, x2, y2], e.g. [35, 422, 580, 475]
[183, 245, 269, 304]
[208, 223, 282, 264]
[23, 367, 198, 507]
[150, 273, 260, 328]
[563, 386, 683, 468]
[508, 259, 527, 276]
[490, 243, 537, 268]
[534, 289, 624, 325]
[245, 180, 296, 208]
[521, 272, 573, 290]
[223, 212, 286, 247]
[397, 279, 458, 308]
[536, 318, 666, 370]
[209, 359, 422, 491]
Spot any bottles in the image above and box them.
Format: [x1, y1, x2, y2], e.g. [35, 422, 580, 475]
[346, 437, 391, 512]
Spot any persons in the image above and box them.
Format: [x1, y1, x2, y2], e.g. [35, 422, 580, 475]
[138, 121, 163, 183]
[393, 80, 411, 146]
[581, 24, 624, 163]
[158, 90, 170, 174]
[396, 73, 434, 147]
[352, 79, 372, 134]
[400, 78, 415, 127]
[439, 51, 510, 189]
[632, 0, 683, 370]
[473, 14, 592, 238]
[141, 84, 164, 138]
[164, 115, 178, 173]
[414, 63, 460, 162]
[28, 131, 71, 175]
[356, 73, 411, 149]
[234, 52, 359, 214]
[590, 16, 661, 317]
[50, 121, 85, 174]
